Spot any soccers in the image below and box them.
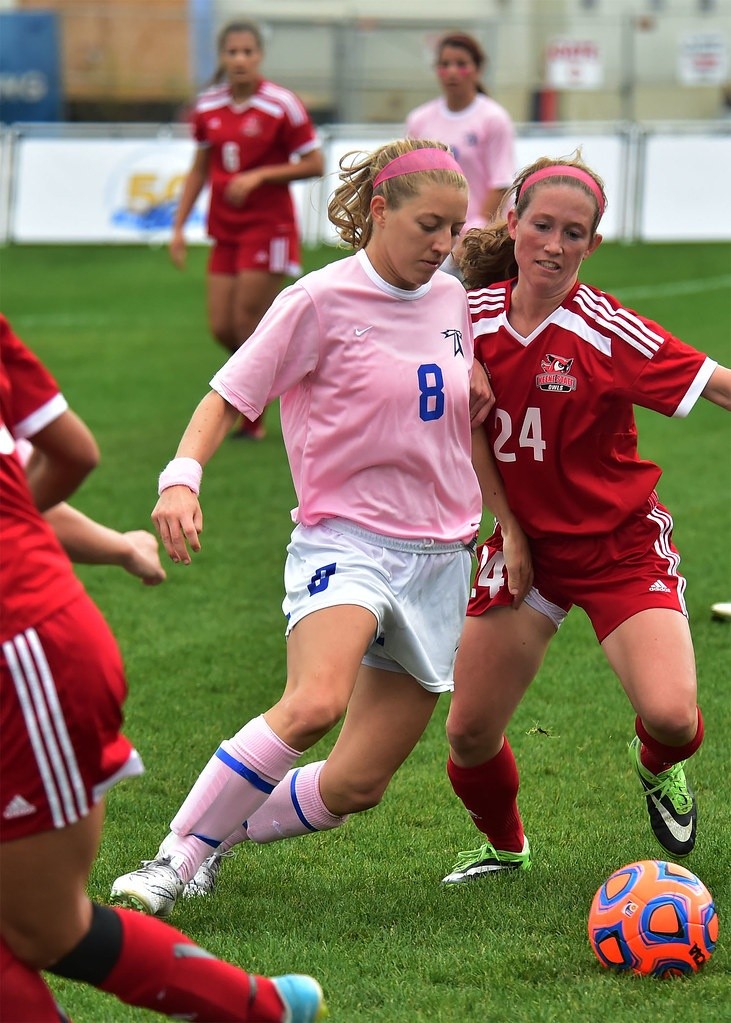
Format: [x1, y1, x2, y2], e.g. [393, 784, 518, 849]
[587, 860, 718, 979]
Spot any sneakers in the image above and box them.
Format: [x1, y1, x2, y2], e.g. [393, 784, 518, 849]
[441, 835, 532, 890]
[107, 848, 185, 922]
[628, 735, 697, 860]
[269, 974, 329, 1023]
[182, 850, 236, 899]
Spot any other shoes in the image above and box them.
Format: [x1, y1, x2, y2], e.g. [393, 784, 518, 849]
[712, 602, 730, 618]
[237, 413, 263, 438]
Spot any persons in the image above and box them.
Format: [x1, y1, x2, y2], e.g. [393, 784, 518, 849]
[406, 33, 516, 290]
[109, 140, 530, 920]
[0, 317, 328, 1023]
[172, 20, 323, 442]
[445, 155, 731, 882]
[14, 439, 164, 591]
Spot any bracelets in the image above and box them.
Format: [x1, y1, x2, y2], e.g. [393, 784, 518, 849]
[158, 457, 204, 496]
